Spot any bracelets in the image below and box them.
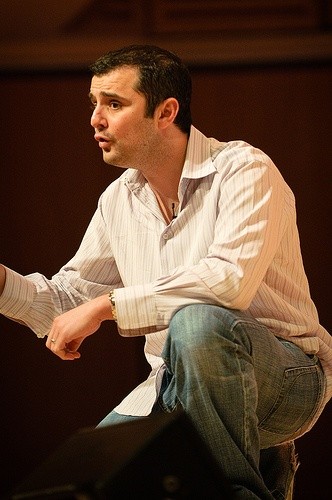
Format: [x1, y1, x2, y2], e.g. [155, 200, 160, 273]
[109, 291, 117, 325]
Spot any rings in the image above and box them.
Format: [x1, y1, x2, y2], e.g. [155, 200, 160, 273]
[48, 338, 57, 344]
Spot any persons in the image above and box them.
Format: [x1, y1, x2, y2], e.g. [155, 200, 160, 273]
[0, 44, 332, 500]
